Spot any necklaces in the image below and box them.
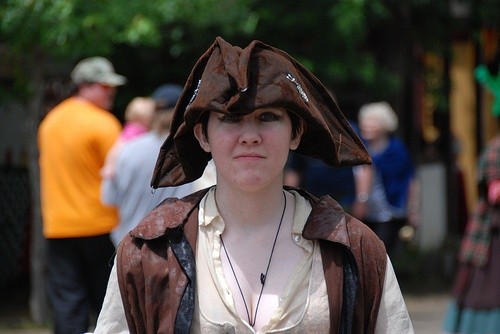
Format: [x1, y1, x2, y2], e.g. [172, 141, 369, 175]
[213, 188, 287, 328]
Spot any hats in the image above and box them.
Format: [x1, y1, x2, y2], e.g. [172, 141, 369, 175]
[72, 56, 126, 88]
[153, 83, 183, 110]
[151, 39, 373, 187]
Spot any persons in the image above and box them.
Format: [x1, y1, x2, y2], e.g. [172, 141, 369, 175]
[443, 137, 500, 334]
[36, 57, 127, 334]
[100, 84, 218, 257]
[285, 102, 410, 262]
[123, 98, 157, 140]
[94, 36, 414, 334]
